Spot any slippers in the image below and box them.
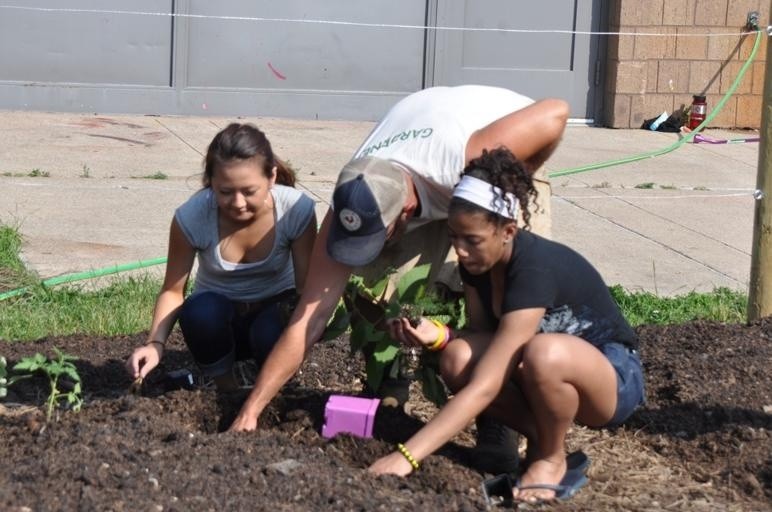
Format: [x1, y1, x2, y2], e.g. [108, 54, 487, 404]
[512, 471, 588, 508]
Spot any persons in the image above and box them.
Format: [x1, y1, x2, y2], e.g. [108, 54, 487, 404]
[123, 123, 321, 397]
[213, 85, 574, 473]
[363, 147, 647, 506]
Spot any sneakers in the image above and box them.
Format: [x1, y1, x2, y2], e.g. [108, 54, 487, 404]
[474, 414, 521, 472]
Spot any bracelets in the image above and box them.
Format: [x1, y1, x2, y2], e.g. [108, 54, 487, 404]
[427, 319, 451, 351]
[397, 442, 420, 470]
[144, 339, 168, 347]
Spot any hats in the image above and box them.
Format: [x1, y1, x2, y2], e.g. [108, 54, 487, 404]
[326, 155, 408, 268]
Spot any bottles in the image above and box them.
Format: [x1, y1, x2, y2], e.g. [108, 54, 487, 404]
[688, 94, 707, 131]
